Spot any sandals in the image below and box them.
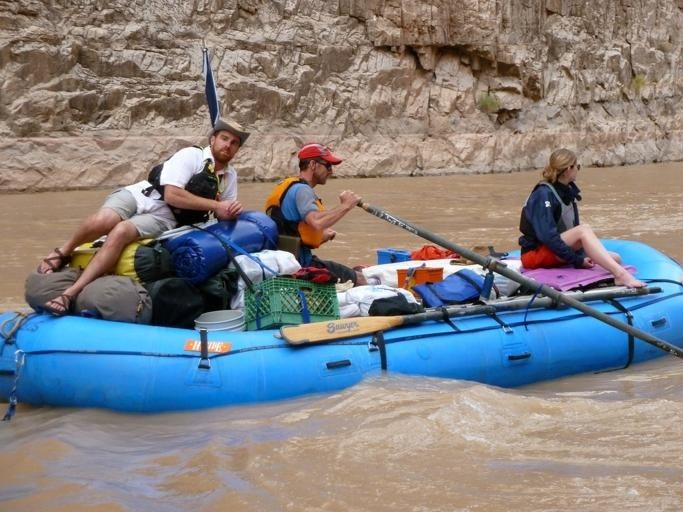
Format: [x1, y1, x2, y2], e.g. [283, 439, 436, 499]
[37, 247, 71, 274]
[44, 292, 74, 313]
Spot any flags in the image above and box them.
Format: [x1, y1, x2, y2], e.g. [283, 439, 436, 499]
[202, 48, 220, 128]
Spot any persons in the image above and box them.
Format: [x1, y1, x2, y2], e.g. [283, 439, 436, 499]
[518, 147, 647, 289]
[263, 143, 368, 287]
[39, 116, 251, 316]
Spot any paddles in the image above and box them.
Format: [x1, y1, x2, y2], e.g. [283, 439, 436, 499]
[280, 285, 662, 345]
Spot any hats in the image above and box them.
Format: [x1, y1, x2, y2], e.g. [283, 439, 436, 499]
[298, 142, 342, 165]
[213, 116, 252, 147]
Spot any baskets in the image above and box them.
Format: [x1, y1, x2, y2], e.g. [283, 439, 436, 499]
[243, 277, 341, 331]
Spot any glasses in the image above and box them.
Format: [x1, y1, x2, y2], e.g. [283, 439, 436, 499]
[576, 162, 580, 171]
[315, 156, 331, 168]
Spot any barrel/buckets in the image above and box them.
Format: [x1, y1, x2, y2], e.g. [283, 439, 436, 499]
[377, 249, 409, 263]
[194, 310, 246, 330]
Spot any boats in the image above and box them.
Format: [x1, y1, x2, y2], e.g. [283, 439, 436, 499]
[0, 241, 682, 412]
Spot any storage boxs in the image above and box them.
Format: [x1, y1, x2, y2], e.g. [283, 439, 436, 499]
[375, 247, 413, 265]
